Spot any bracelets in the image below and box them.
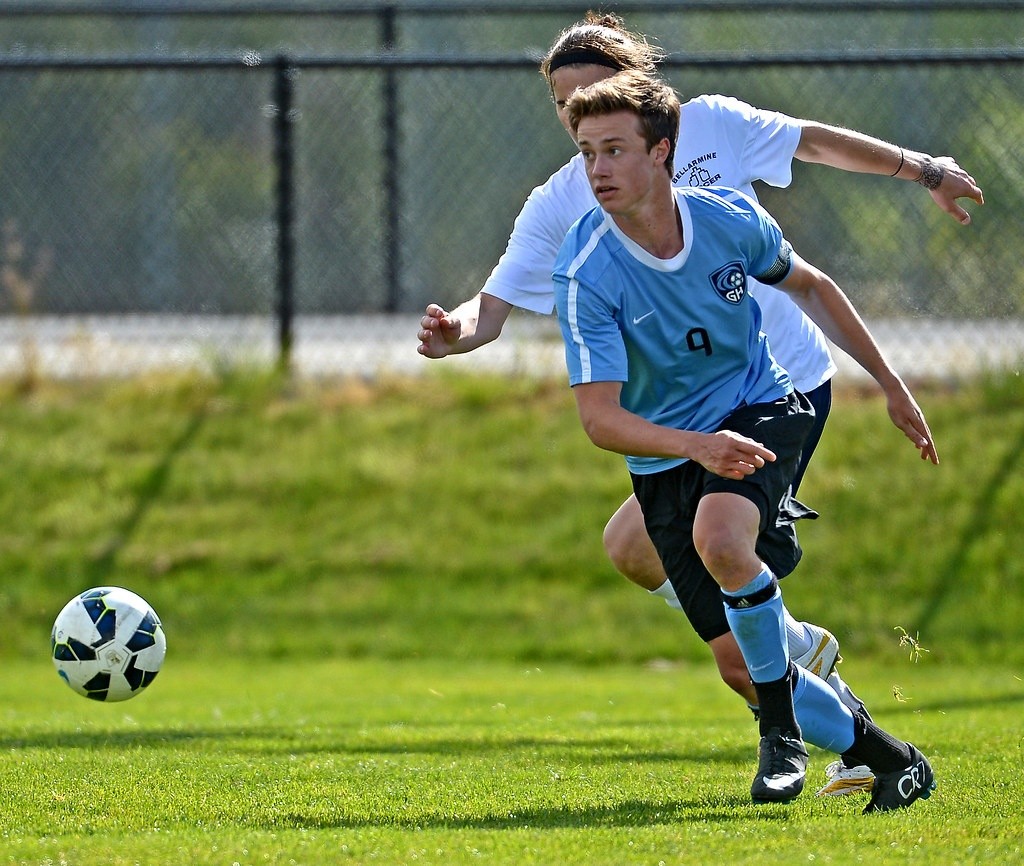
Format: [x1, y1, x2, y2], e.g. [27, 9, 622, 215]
[890, 148, 904, 177]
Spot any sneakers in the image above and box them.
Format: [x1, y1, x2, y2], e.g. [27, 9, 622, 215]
[813, 761, 874, 798]
[861, 742, 937, 815]
[750, 727, 809, 803]
[792, 621, 843, 681]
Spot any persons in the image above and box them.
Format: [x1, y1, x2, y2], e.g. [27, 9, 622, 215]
[550, 70, 939, 815]
[416, 10, 983, 796]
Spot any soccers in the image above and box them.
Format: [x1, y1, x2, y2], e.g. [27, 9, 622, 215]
[50, 585, 168, 703]
